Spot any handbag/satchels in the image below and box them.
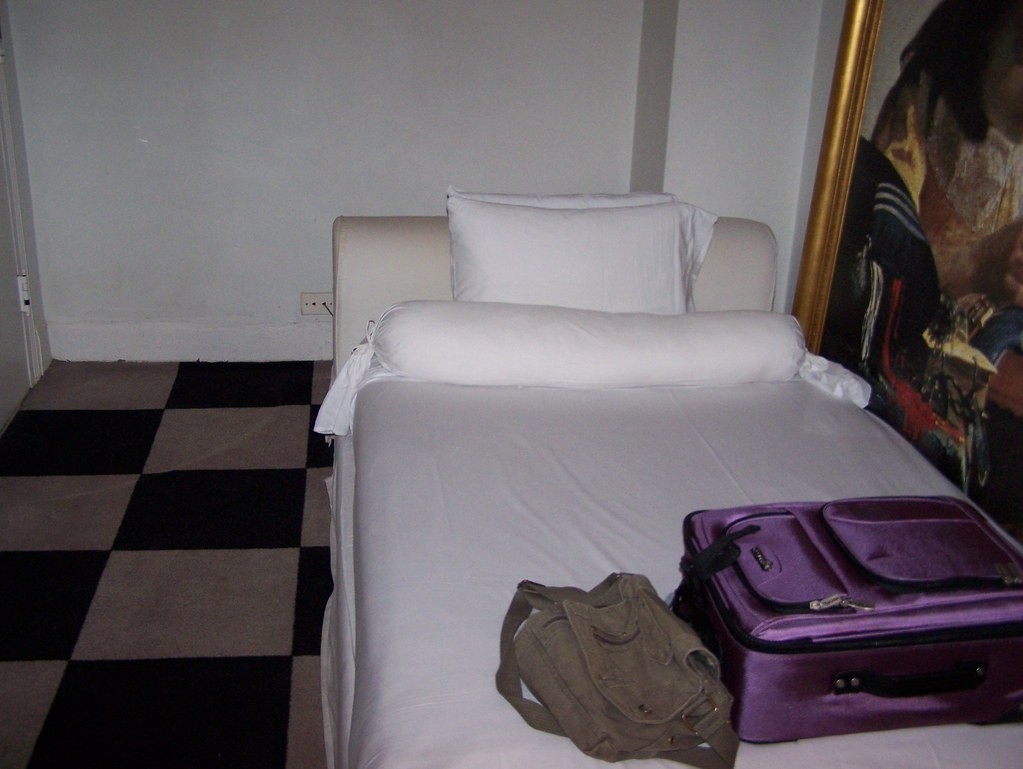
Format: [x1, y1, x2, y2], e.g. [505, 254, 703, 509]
[496, 572, 740, 769]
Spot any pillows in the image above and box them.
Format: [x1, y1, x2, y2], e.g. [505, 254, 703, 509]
[313, 301, 871, 436]
[447, 196, 717, 317]
[447, 185, 674, 210]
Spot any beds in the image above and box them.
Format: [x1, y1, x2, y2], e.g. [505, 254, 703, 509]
[321, 215, 1023, 769]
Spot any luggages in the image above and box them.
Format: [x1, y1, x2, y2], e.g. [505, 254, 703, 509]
[671, 496, 1023, 744]
